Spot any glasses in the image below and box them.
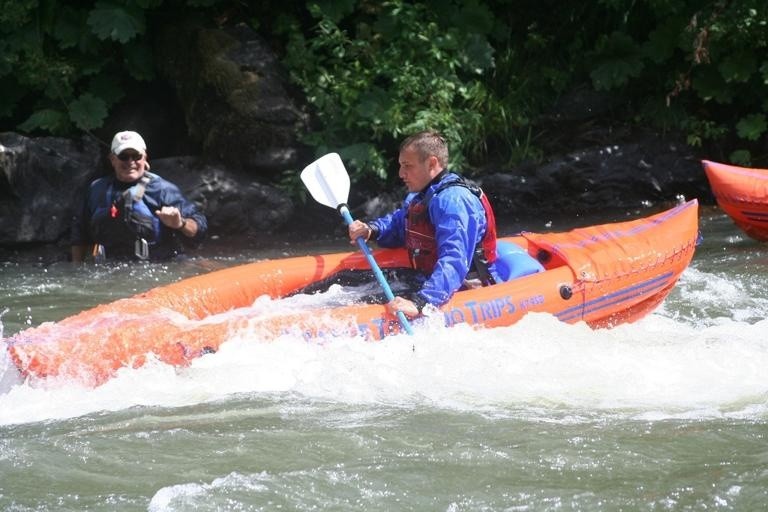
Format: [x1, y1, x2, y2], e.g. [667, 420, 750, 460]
[117, 152, 142, 161]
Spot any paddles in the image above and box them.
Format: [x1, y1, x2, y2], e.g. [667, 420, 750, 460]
[300, 153, 414, 336]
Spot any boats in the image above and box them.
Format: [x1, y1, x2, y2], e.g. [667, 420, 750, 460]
[701, 160, 768, 242]
[1, 198, 700, 390]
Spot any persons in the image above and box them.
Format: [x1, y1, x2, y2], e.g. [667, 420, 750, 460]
[280, 129, 497, 318]
[68, 130, 209, 264]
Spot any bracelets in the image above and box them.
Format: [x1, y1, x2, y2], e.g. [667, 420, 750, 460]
[179, 218, 186, 230]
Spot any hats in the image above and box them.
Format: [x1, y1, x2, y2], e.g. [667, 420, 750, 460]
[110, 130, 146, 155]
